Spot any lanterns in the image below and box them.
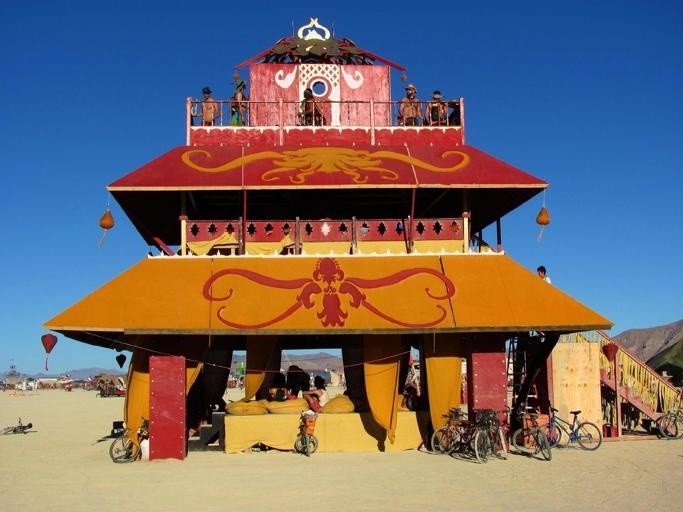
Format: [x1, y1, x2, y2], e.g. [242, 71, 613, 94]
[41, 334, 58, 371]
[536, 208, 550, 241]
[116, 354, 126, 368]
[98, 209, 114, 247]
[602, 343, 618, 379]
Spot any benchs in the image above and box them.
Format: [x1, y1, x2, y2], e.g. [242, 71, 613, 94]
[186, 243, 302, 256]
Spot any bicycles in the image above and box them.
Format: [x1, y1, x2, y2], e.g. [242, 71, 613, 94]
[0, 417, 32, 436]
[430, 400, 601, 465]
[109, 416, 149, 464]
[294, 410, 318, 458]
[658, 390, 682, 440]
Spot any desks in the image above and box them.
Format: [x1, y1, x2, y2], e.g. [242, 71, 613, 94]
[224, 408, 431, 453]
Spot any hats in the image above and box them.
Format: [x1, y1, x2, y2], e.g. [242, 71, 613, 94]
[236, 80, 247, 92]
[405, 84, 417, 93]
[203, 87, 211, 94]
[432, 91, 444, 99]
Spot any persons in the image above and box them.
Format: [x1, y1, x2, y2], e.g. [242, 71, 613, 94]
[537, 266, 552, 284]
[469, 236, 478, 253]
[399, 84, 423, 126]
[298, 88, 327, 126]
[424, 91, 458, 126]
[230, 80, 248, 126]
[192, 87, 220, 126]
[255, 365, 329, 414]
